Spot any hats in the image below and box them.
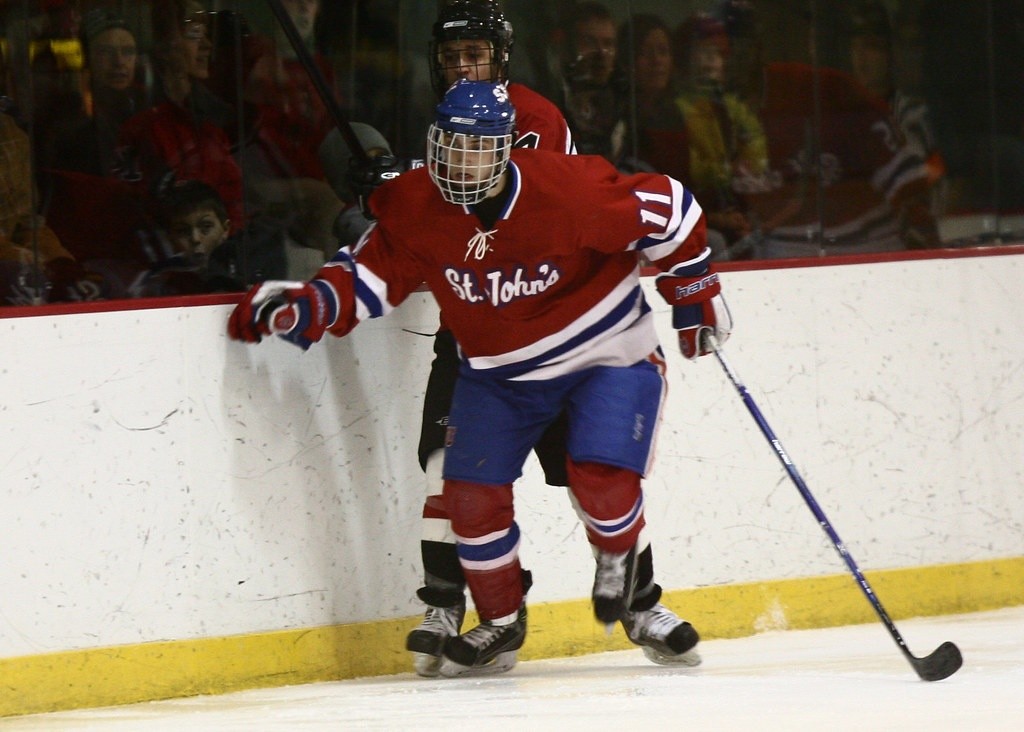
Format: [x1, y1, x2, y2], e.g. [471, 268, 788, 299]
[81, 9, 138, 45]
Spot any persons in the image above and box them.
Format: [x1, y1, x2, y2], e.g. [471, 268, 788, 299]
[405, 0, 581, 681]
[1, 0, 1023, 310]
[227, 80, 729, 679]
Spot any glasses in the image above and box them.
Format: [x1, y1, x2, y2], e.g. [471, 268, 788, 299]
[181, 19, 210, 40]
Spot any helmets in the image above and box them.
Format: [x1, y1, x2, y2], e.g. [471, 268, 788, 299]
[427, 77, 517, 204]
[431, 1, 513, 78]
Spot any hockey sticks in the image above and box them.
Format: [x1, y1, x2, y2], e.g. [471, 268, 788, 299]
[705, 328, 965, 683]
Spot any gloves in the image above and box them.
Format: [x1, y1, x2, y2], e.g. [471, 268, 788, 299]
[226, 279, 331, 353]
[656, 247, 733, 358]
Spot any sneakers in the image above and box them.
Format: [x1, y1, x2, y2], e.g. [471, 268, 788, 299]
[620, 584, 701, 667]
[592, 543, 634, 634]
[405, 583, 466, 677]
[440, 568, 533, 677]
